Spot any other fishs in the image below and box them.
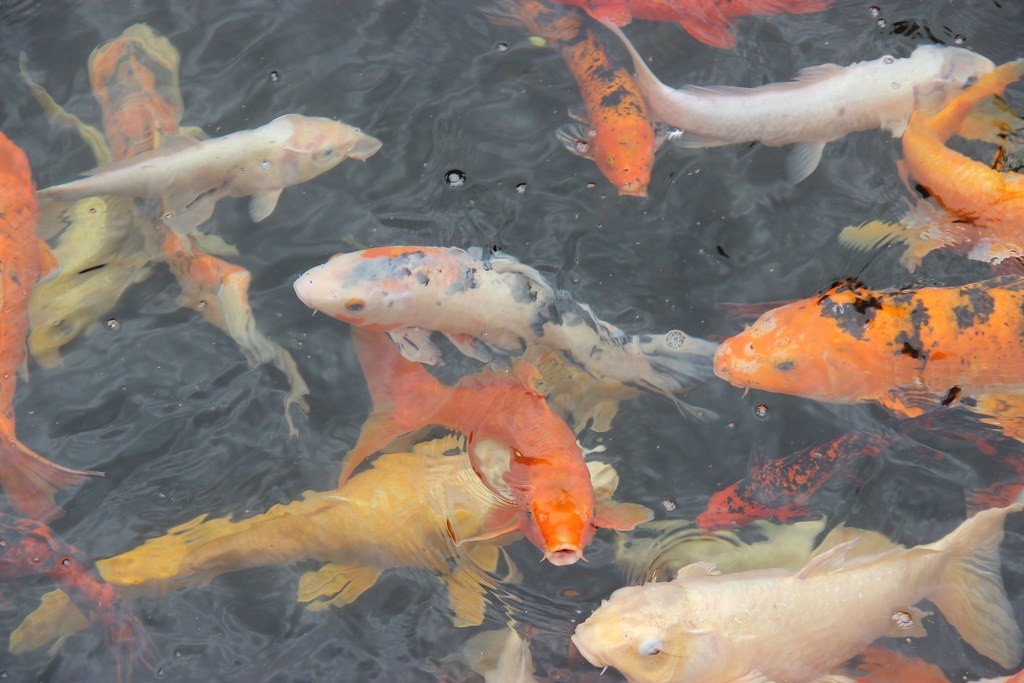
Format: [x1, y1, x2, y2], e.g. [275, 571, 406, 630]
[0, 1, 1024, 682]
